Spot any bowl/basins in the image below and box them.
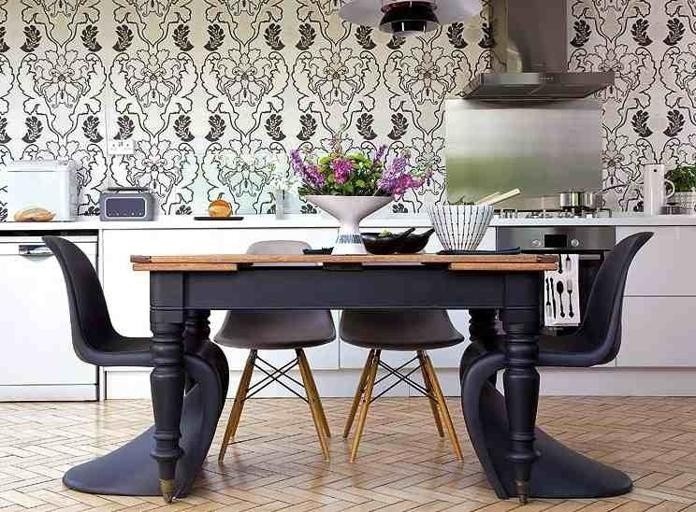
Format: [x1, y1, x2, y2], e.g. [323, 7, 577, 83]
[428, 205, 495, 250]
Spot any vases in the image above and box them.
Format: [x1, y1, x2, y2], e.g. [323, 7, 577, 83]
[303, 194, 391, 256]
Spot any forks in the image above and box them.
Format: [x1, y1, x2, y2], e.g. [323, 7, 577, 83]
[565, 255, 571, 270]
[545, 278, 551, 317]
[566, 280, 574, 318]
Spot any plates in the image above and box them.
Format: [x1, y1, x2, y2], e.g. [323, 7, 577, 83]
[195, 216, 244, 221]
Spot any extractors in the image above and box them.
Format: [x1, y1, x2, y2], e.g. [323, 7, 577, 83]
[461, 0, 614, 103]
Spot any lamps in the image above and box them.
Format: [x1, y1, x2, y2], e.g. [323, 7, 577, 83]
[339, 0, 483, 35]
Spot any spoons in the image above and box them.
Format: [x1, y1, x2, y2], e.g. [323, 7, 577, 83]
[556, 281, 564, 318]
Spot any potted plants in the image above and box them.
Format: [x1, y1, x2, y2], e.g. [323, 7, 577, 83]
[666, 166, 695, 213]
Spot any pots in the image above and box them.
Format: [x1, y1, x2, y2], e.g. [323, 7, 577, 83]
[556, 184, 629, 207]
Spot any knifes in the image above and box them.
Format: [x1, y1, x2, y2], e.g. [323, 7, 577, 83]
[549, 278, 556, 318]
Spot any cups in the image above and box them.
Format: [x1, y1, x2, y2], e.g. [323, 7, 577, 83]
[644, 163, 675, 215]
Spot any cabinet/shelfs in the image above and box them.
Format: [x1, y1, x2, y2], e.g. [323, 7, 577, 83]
[614, 227, 695, 370]
[102, 231, 339, 371]
[339, 230, 615, 370]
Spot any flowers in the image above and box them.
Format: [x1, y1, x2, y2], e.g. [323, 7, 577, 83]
[291, 145, 425, 200]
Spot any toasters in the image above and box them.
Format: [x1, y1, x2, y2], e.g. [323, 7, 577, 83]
[99, 185, 152, 220]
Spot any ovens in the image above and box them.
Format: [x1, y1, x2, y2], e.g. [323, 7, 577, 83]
[497, 226, 615, 340]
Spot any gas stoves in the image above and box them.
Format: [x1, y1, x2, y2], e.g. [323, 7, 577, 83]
[496, 209, 613, 218]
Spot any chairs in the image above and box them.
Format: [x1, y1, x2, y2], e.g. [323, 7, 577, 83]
[41, 235, 229, 497]
[459, 232, 655, 499]
[213, 240, 336, 463]
[339, 309, 465, 463]
